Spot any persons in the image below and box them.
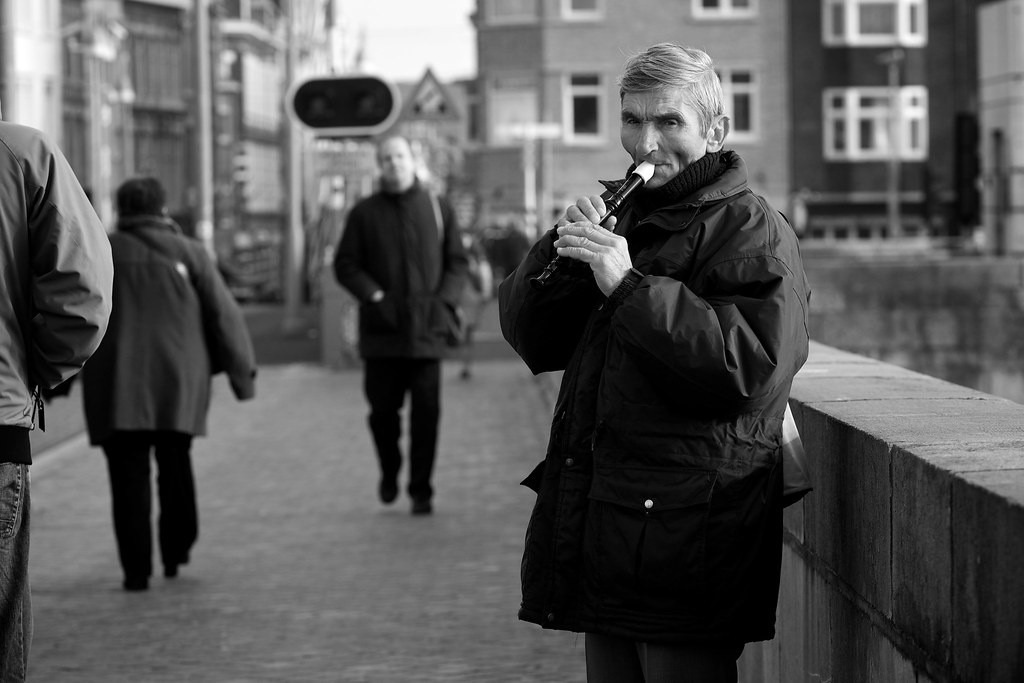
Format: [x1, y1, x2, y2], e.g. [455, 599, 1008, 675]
[498, 41, 812, 683]
[497, 221, 533, 281]
[39, 176, 255, 591]
[335, 133, 471, 515]
[0, 124, 115, 683]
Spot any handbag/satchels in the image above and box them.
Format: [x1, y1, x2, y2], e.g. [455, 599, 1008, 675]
[782, 401, 814, 510]
[447, 274, 483, 348]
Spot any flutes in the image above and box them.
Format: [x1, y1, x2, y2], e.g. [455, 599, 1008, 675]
[530, 161, 655, 294]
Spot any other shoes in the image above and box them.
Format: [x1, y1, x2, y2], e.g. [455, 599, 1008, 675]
[163, 557, 179, 576]
[411, 496, 431, 513]
[124, 574, 148, 590]
[379, 456, 402, 503]
